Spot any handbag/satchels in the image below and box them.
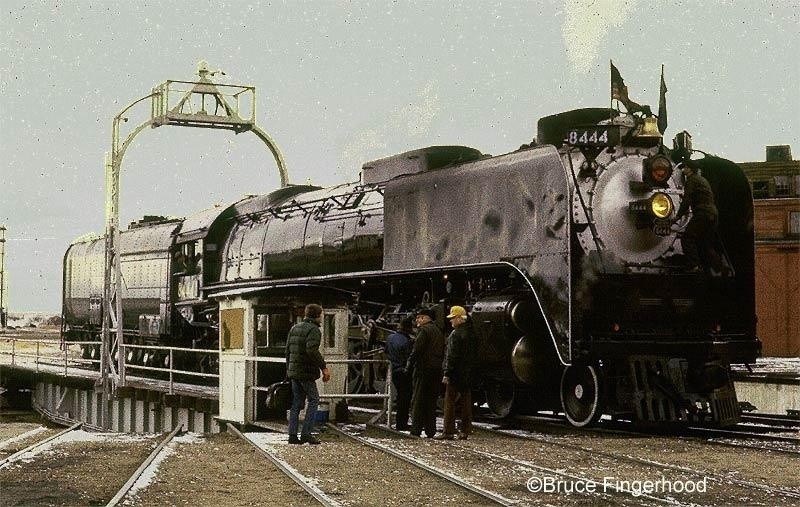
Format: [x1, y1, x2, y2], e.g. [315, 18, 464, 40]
[265, 378, 306, 411]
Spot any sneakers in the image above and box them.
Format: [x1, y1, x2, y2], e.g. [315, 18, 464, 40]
[301, 436, 321, 445]
[433, 433, 454, 440]
[396, 425, 436, 438]
[289, 438, 302, 444]
[457, 431, 468, 440]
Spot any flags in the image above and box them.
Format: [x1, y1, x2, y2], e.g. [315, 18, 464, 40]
[657, 75, 667, 135]
[611, 63, 643, 115]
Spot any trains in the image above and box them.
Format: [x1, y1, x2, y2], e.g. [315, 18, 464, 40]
[61, 50, 767, 439]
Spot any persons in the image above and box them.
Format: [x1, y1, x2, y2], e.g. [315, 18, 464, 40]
[405, 308, 444, 439]
[670, 160, 733, 276]
[384, 319, 416, 434]
[284, 303, 330, 444]
[432, 305, 477, 440]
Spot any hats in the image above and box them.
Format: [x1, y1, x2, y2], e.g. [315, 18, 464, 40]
[446, 305, 467, 318]
[413, 308, 437, 321]
[305, 303, 323, 319]
